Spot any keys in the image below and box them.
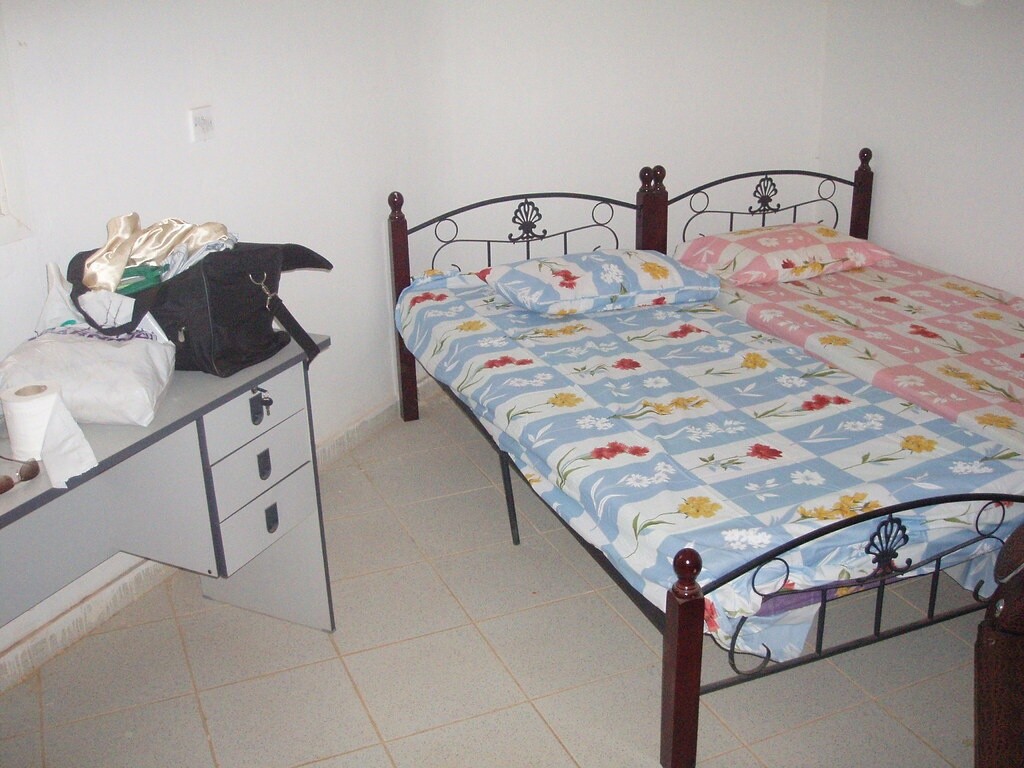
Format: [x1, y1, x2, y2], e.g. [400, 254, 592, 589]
[257, 386, 273, 416]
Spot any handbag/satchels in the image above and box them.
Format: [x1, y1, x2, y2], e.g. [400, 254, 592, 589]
[66, 242, 333, 379]
[0, 261, 177, 429]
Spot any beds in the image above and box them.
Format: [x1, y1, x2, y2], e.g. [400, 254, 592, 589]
[388, 147, 1023, 768]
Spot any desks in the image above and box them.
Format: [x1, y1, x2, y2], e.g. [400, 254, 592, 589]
[1, 333, 337, 633]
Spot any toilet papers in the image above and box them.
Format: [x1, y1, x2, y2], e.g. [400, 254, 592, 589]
[2, 379, 98, 492]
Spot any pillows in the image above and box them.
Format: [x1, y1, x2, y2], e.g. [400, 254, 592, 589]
[475, 251, 720, 316]
[0, 333, 177, 427]
[673, 222, 869, 286]
